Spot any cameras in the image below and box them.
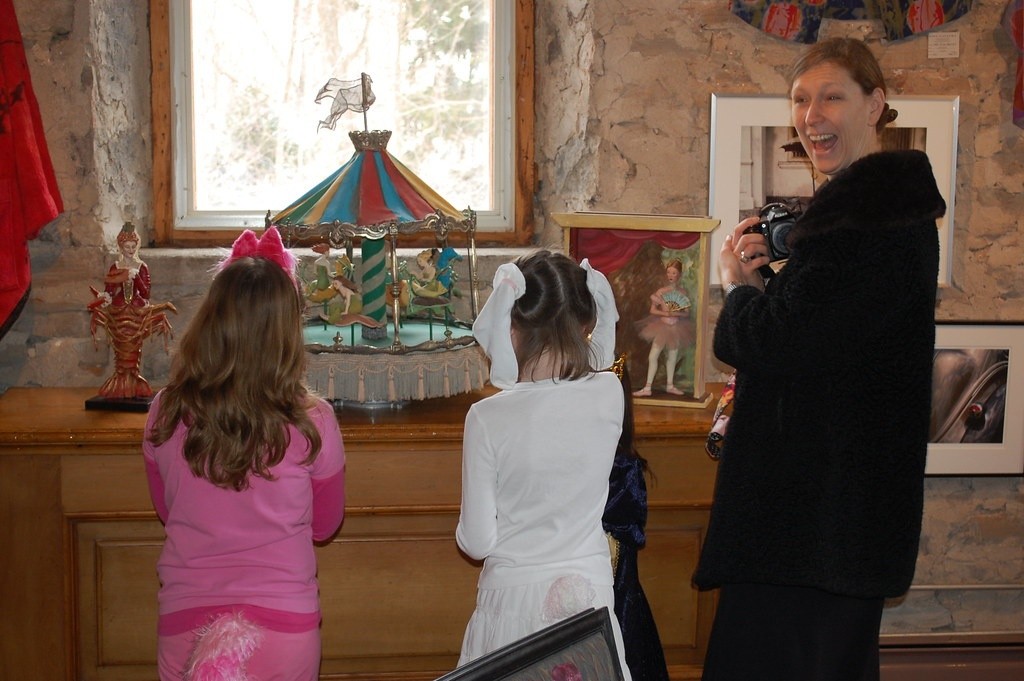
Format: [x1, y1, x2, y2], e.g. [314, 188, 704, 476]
[739, 199, 804, 267]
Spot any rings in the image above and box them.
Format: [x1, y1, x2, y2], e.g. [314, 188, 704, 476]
[741, 251, 750, 263]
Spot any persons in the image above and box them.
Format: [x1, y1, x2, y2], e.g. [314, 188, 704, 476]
[105, 223, 150, 307]
[690, 38, 946, 681]
[455, 250, 632, 681]
[314, 245, 336, 288]
[144, 257, 347, 681]
[411, 249, 444, 291]
[320, 276, 362, 323]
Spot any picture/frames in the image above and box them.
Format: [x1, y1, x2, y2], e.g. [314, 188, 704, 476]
[549, 209, 723, 409]
[707, 92, 960, 289]
[924, 319, 1024, 479]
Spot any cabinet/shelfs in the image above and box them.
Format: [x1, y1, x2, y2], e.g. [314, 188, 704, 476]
[0, 384, 729, 681]
